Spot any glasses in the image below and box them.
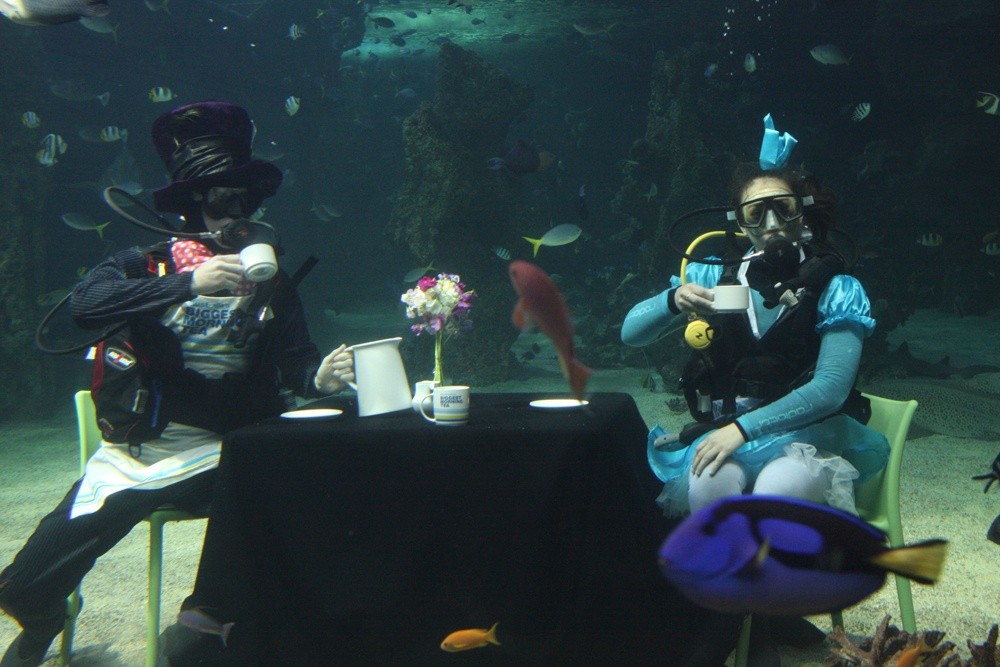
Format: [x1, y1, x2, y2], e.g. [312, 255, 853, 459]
[731, 191, 806, 231]
[192, 185, 263, 222]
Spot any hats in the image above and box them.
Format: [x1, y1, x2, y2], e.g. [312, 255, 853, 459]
[145, 96, 284, 221]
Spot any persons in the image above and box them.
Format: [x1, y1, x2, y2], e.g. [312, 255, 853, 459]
[1, 99, 359, 667]
[618, 112, 875, 647]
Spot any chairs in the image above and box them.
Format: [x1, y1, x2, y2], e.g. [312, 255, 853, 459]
[54, 387, 212, 667]
[731, 393, 918, 666]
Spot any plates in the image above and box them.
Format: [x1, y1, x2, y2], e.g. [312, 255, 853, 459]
[529, 399, 590, 411]
[280, 408, 343, 422]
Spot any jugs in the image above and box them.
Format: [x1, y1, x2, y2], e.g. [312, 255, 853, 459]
[341, 337, 414, 418]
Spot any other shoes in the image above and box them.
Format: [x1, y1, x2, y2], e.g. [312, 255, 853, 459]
[1, 590, 83, 666]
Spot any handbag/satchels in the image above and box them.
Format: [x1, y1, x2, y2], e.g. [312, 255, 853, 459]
[85, 293, 185, 447]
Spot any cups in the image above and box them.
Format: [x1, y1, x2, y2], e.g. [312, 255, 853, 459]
[710, 284, 749, 313]
[419, 386, 471, 427]
[412, 381, 441, 414]
[239, 243, 279, 282]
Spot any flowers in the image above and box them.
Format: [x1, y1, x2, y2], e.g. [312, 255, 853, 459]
[400, 272, 480, 386]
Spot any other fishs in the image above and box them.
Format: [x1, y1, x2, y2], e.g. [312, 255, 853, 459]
[172, 606, 236, 646]
[888, 231, 1000, 259]
[654, 494, 950, 620]
[438, 623, 500, 653]
[490, 223, 592, 401]
[489, 0, 998, 176]
[972, 453, 999, 494]
[1, 0, 488, 308]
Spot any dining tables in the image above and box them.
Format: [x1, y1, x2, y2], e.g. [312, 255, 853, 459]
[170, 387, 685, 665]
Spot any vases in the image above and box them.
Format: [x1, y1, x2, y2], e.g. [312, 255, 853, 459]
[432, 334, 444, 390]
